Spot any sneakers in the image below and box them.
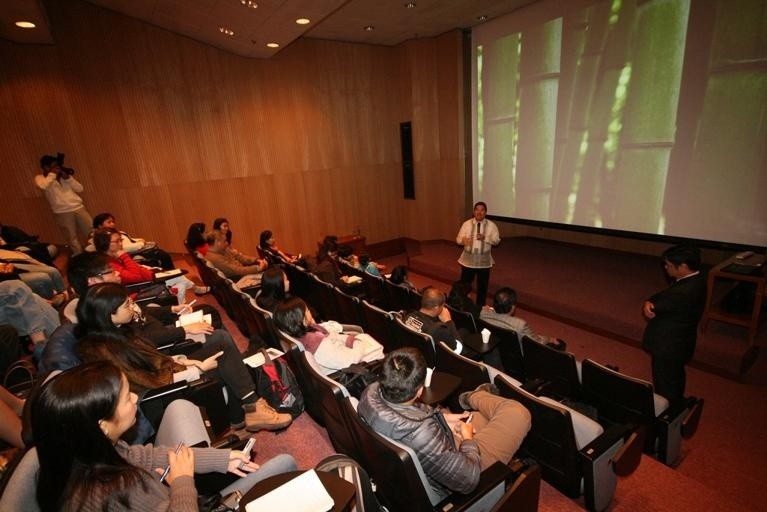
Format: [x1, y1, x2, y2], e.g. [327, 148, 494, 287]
[242, 397, 293, 432]
[458, 383, 500, 410]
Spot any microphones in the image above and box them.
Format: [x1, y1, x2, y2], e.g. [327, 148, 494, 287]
[477, 222, 480, 240]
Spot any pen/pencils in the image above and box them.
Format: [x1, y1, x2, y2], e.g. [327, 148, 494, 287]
[465, 414, 473, 424]
[159, 441, 184, 484]
[177, 299, 197, 315]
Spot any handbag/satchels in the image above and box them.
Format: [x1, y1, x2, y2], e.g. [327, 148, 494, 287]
[340, 368, 371, 396]
[4, 359, 39, 401]
[241, 344, 304, 421]
[315, 453, 387, 511]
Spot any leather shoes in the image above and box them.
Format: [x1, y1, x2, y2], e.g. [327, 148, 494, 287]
[195, 286, 218, 296]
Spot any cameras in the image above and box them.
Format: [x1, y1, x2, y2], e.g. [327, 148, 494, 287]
[57, 152, 74, 175]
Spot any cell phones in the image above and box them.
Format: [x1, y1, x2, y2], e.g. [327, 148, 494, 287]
[455, 411, 473, 433]
[234, 437, 256, 469]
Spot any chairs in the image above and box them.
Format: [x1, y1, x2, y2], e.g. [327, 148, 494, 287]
[0, 231, 704, 512]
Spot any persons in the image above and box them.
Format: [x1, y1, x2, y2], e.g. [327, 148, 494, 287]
[36, 156, 93, 255]
[641, 244, 706, 407]
[455, 202, 501, 312]
[1, 213, 293, 512]
[276, 235, 566, 512]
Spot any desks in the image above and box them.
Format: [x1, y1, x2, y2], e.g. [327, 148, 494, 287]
[703, 251, 767, 348]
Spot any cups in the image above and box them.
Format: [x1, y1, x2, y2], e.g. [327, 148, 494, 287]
[424, 368, 432, 388]
[481, 331, 491, 344]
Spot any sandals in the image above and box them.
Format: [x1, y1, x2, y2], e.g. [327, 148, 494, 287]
[47, 285, 78, 309]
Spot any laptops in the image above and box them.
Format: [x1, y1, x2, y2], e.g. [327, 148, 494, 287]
[720, 259, 767, 274]
